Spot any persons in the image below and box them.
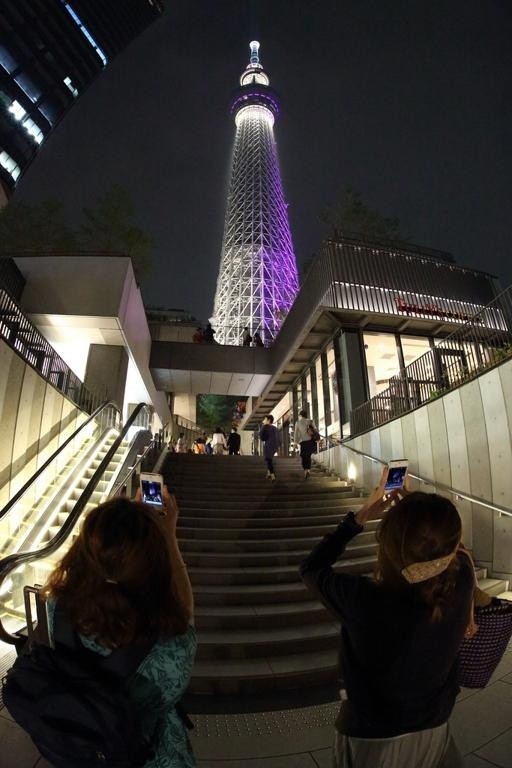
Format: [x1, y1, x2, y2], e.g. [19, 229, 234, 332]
[257, 413, 282, 483]
[201, 322, 219, 344]
[192, 326, 204, 344]
[253, 332, 265, 347]
[173, 424, 242, 456]
[290, 408, 320, 479]
[296, 462, 477, 767]
[240, 326, 251, 347]
[31, 482, 203, 766]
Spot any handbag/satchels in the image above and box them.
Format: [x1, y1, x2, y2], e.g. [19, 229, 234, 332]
[306, 419, 321, 440]
[455, 545, 512, 688]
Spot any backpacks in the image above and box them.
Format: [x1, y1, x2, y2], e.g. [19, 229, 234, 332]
[1, 576, 167, 768]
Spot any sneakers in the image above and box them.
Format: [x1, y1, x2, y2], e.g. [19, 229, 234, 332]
[262, 466, 312, 482]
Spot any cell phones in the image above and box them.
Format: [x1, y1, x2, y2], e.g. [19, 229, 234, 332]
[384, 458, 409, 495]
[139, 471, 164, 511]
